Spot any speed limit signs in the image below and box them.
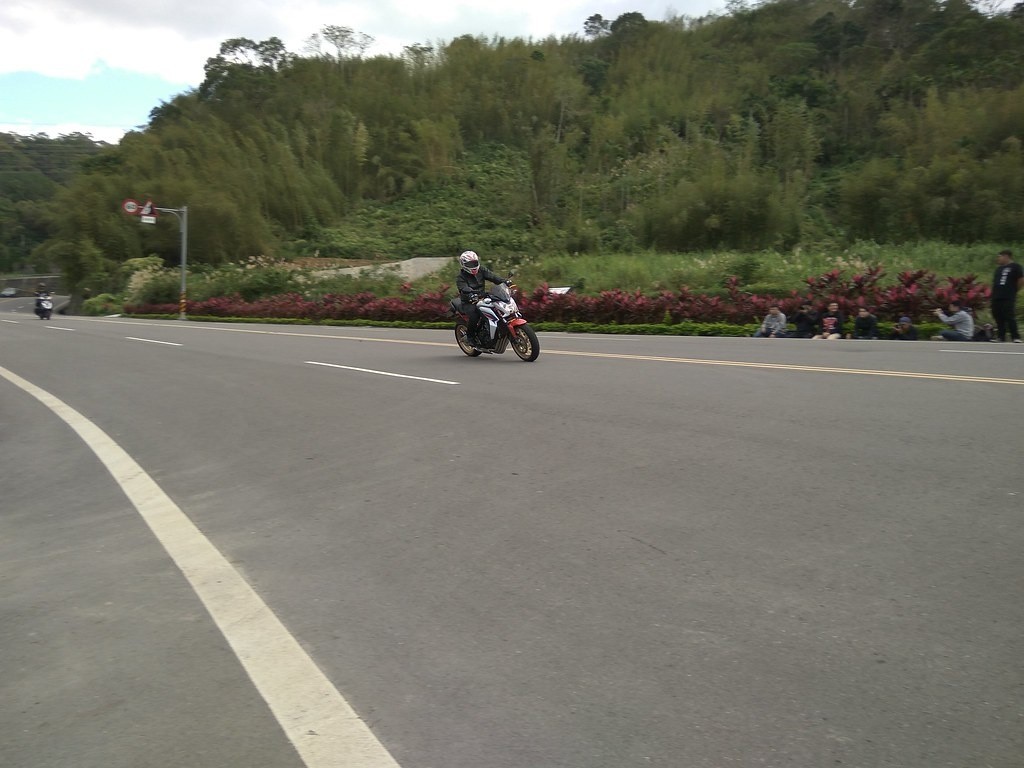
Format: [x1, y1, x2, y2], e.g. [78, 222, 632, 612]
[122, 200, 139, 214]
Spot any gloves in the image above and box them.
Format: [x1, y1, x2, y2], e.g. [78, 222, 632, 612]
[470, 294, 478, 304]
[505, 280, 513, 287]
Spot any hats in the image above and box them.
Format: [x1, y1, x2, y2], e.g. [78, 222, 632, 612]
[899, 317, 912, 325]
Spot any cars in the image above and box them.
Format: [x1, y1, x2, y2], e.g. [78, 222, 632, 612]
[1, 287, 18, 297]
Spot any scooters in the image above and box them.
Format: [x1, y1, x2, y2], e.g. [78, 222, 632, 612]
[33, 291, 56, 320]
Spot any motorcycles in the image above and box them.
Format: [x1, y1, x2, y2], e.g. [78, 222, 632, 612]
[446, 272, 540, 363]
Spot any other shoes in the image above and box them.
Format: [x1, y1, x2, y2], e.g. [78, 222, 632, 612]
[1013, 338, 1023, 343]
[468, 340, 477, 347]
[930, 334, 945, 341]
[990, 339, 1006, 343]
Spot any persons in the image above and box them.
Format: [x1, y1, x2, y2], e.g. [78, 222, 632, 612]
[457, 251, 513, 348]
[990, 250, 1024, 343]
[34, 283, 54, 307]
[755, 304, 786, 338]
[853, 308, 880, 340]
[889, 317, 918, 340]
[777, 301, 818, 338]
[933, 302, 975, 341]
[811, 302, 843, 339]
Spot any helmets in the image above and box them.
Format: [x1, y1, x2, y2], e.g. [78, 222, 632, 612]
[38, 282, 47, 289]
[459, 251, 480, 274]
